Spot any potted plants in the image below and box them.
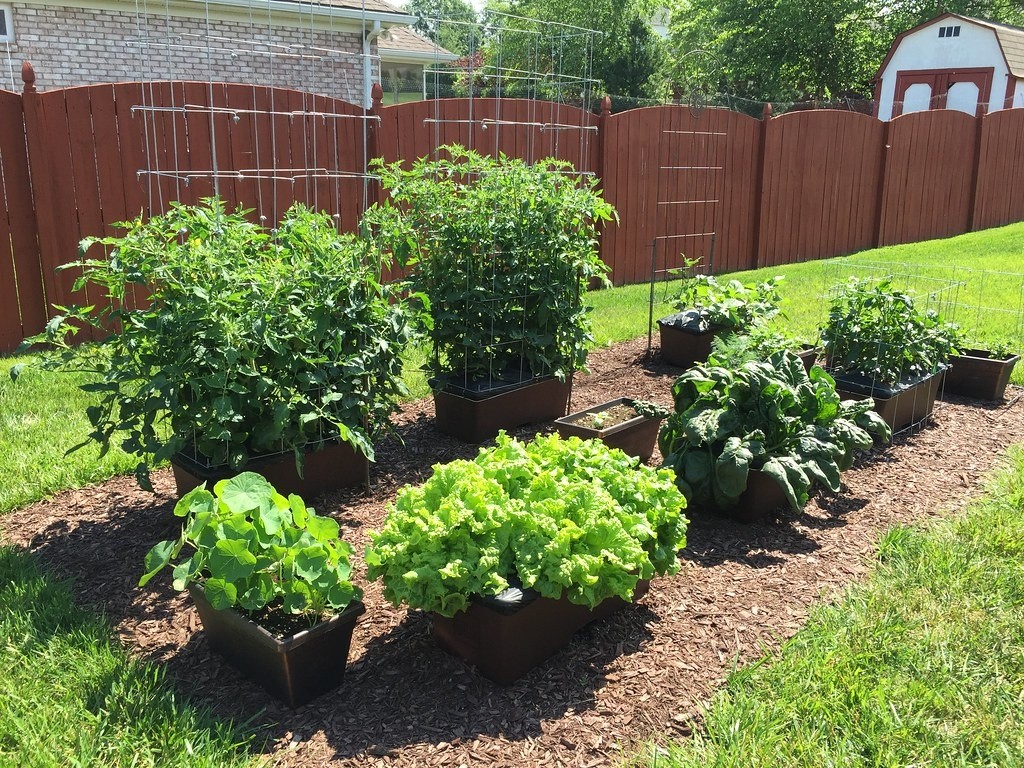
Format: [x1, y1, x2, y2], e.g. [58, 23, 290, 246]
[658, 351, 892, 521]
[139, 474, 363, 708]
[13, 198, 437, 488]
[654, 252, 789, 367]
[815, 279, 940, 440]
[554, 399, 670, 464]
[369, 141, 618, 442]
[940, 342, 1021, 405]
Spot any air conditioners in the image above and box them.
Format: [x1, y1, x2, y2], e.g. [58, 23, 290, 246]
[367, 423, 692, 686]
